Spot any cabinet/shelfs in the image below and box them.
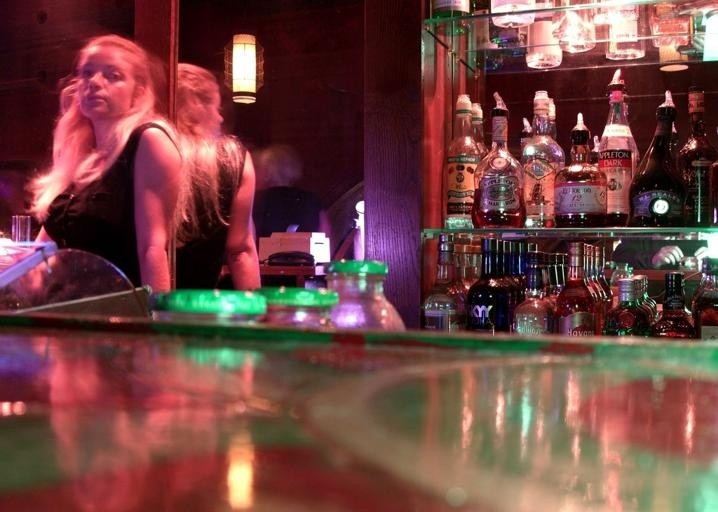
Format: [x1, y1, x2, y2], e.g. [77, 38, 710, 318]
[423, 0, 718, 234]
[221, 265, 314, 288]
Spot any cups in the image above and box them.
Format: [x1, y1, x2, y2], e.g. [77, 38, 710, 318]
[10, 214, 31, 243]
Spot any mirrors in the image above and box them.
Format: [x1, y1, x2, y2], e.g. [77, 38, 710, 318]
[168, 5, 363, 292]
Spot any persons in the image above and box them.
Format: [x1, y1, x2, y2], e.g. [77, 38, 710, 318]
[173, 60, 262, 290]
[251, 143, 333, 255]
[21, 33, 184, 293]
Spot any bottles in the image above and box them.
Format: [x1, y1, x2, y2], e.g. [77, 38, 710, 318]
[628, 104, 683, 228]
[657, 47, 690, 72]
[489, 0, 538, 29]
[590, 149, 601, 167]
[422, 234, 718, 340]
[552, 0, 597, 56]
[605, 0, 647, 61]
[498, 25, 527, 59]
[487, 0, 520, 48]
[547, 97, 557, 141]
[646, 2, 690, 49]
[679, 84, 718, 227]
[471, 102, 490, 161]
[555, 129, 607, 227]
[444, 95, 483, 229]
[520, 128, 533, 166]
[471, 108, 526, 229]
[321, 258, 407, 334]
[428, 0, 475, 37]
[526, 0, 564, 69]
[521, 90, 567, 228]
[596, 83, 642, 229]
[476, 0, 501, 75]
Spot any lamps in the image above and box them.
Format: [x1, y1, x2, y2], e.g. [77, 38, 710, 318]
[232, 34, 257, 104]
